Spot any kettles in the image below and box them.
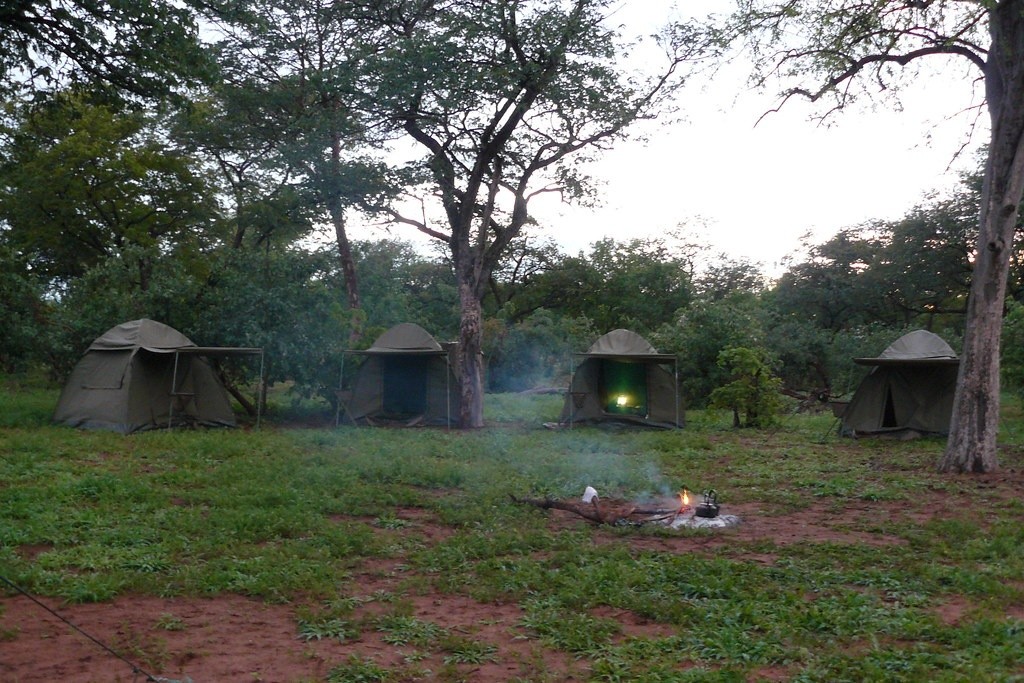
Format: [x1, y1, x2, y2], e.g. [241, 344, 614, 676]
[695, 493, 718, 518]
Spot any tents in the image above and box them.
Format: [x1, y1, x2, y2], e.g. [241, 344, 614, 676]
[561, 330, 684, 432]
[831, 329, 960, 439]
[50, 318, 265, 432]
[336, 324, 463, 432]
